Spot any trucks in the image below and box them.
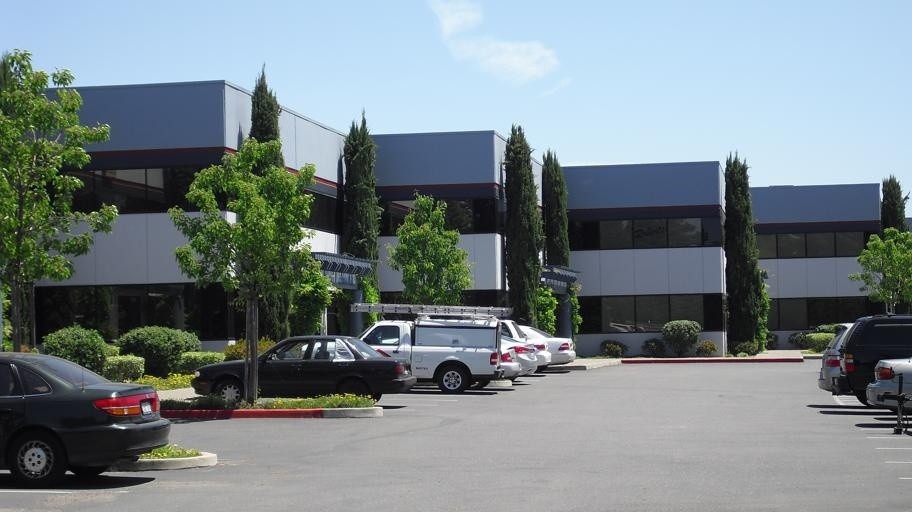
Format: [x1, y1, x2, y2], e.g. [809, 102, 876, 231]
[356, 313, 502, 394]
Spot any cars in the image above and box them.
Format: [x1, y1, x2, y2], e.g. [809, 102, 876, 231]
[191, 336, 417, 404]
[0, 352, 170, 487]
[500, 319, 576, 382]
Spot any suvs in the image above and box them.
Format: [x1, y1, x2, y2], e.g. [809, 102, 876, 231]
[818, 314, 912, 414]
